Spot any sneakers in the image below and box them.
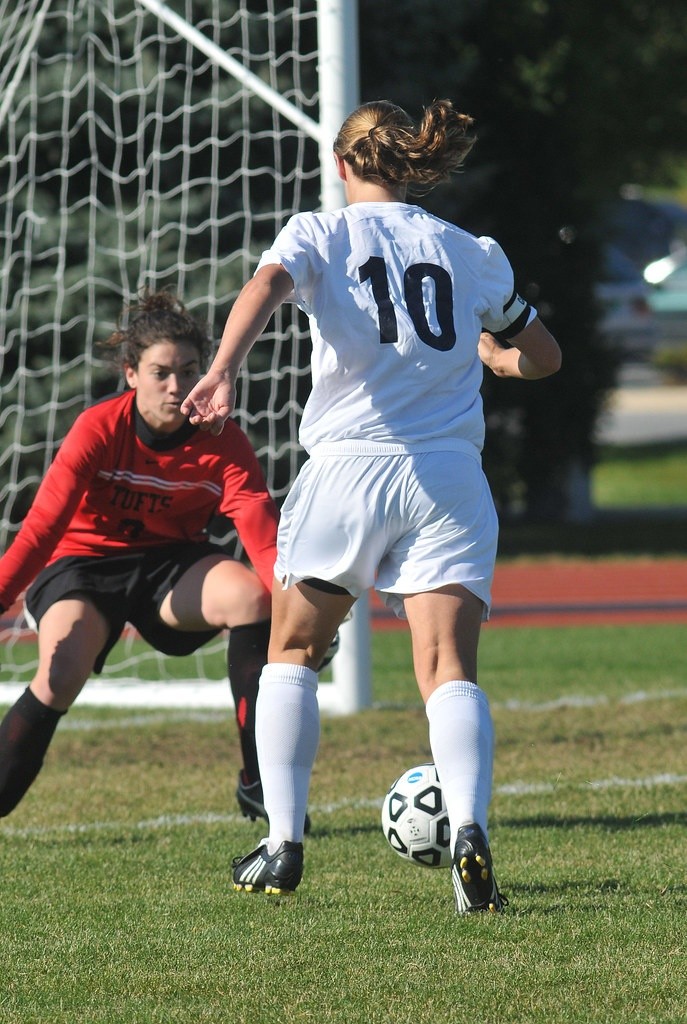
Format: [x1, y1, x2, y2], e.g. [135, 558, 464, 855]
[231, 837, 304, 897]
[235, 769, 311, 834]
[449, 820, 510, 915]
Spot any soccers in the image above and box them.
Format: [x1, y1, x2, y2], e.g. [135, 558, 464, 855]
[383, 762, 451, 868]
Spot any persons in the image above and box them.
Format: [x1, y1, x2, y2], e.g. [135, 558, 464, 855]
[1, 290, 311, 834]
[180, 100, 562, 918]
